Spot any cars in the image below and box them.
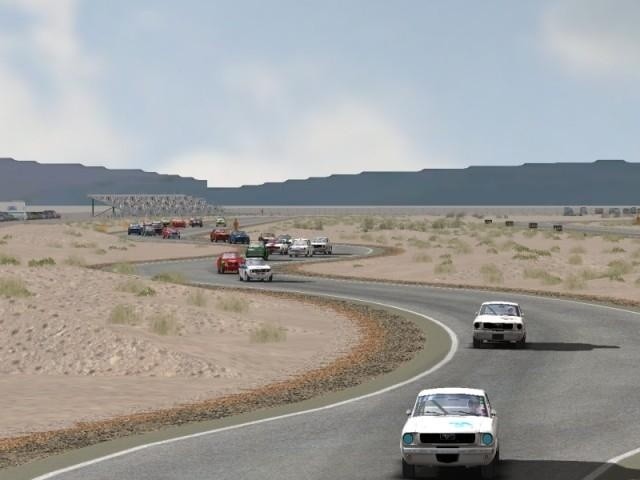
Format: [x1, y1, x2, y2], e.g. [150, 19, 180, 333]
[216, 216, 226, 227]
[128, 216, 202, 239]
[473, 301, 526, 348]
[211, 228, 333, 282]
[400, 387, 500, 478]
